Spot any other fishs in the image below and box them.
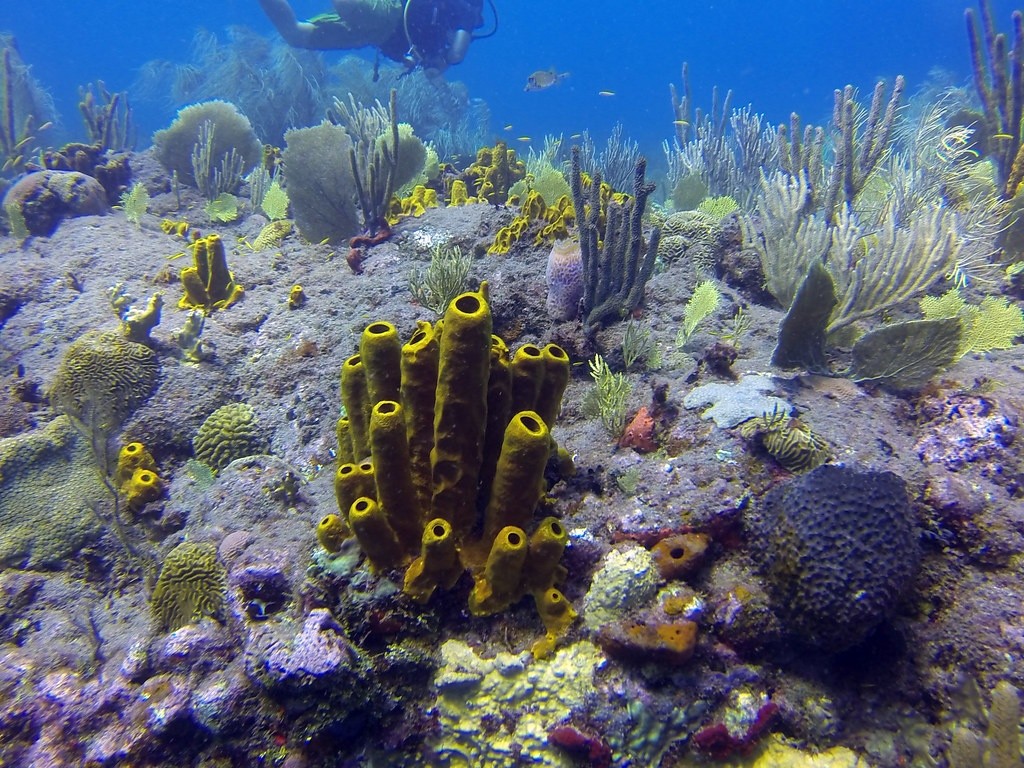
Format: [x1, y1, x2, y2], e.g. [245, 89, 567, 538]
[1, 65, 1024, 179]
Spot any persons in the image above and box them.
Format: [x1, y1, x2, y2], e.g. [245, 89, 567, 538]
[259, 0, 484, 80]
